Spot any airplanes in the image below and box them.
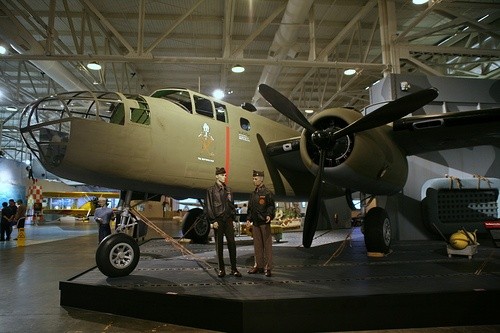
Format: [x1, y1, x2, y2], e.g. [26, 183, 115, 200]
[41, 192, 122, 223]
[19, 83, 500, 279]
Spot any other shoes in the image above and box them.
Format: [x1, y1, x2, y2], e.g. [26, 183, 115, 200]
[231, 269, 241, 277]
[218, 268, 224, 276]
[247, 268, 259, 273]
[264, 269, 271, 276]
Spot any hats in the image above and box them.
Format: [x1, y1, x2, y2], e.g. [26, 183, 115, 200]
[216, 167, 226, 175]
[253, 170, 264, 176]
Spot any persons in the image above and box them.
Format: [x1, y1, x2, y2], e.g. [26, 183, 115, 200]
[34, 199, 42, 225]
[94, 199, 115, 243]
[0, 202, 14, 241]
[246, 170, 276, 277]
[7, 199, 17, 239]
[15, 199, 26, 240]
[207, 167, 242, 277]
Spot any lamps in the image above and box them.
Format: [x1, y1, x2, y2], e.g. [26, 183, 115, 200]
[87, 60, 102, 71]
[231, 64, 245, 73]
